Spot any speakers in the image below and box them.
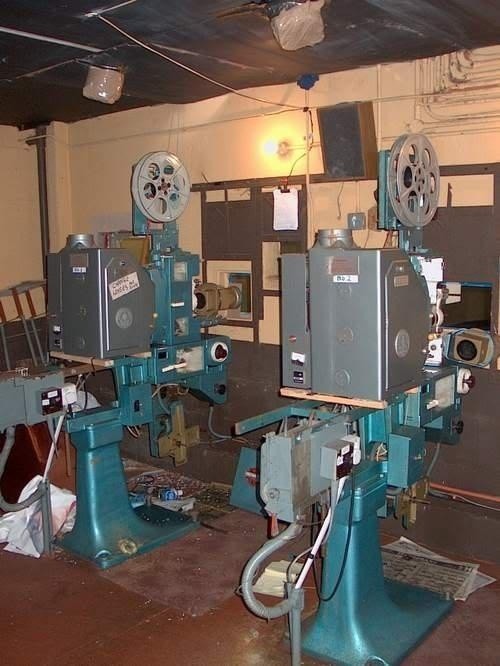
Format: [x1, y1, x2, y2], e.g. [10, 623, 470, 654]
[317, 100, 378, 182]
